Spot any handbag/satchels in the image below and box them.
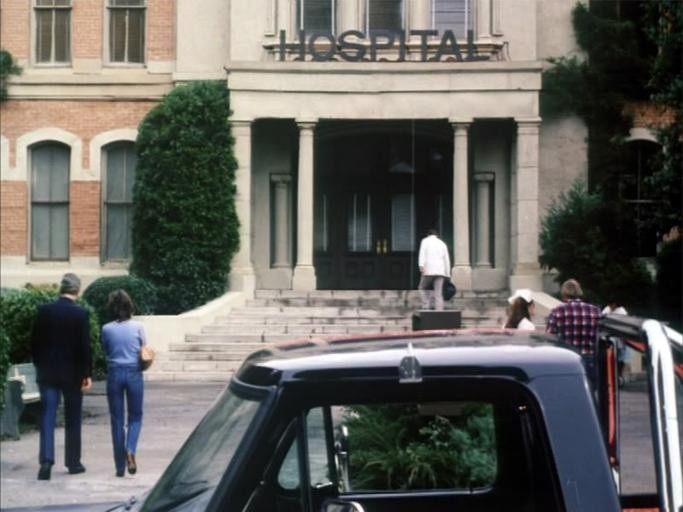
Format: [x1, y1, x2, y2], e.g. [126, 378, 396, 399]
[141, 344, 155, 371]
[443, 279, 458, 301]
[126, 448, 137, 474]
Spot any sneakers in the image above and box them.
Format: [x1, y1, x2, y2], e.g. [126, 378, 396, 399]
[38, 462, 85, 480]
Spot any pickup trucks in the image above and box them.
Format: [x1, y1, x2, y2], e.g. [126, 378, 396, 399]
[1, 313, 683, 512]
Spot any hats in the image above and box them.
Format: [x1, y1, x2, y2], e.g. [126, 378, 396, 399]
[506, 288, 534, 306]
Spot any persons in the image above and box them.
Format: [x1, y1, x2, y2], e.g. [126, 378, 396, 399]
[417, 228, 449, 310]
[30, 271, 92, 480]
[99, 289, 145, 476]
[546, 278, 600, 400]
[601, 295, 628, 386]
[502, 287, 537, 331]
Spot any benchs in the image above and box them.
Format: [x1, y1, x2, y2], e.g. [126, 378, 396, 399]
[0, 363, 43, 440]
